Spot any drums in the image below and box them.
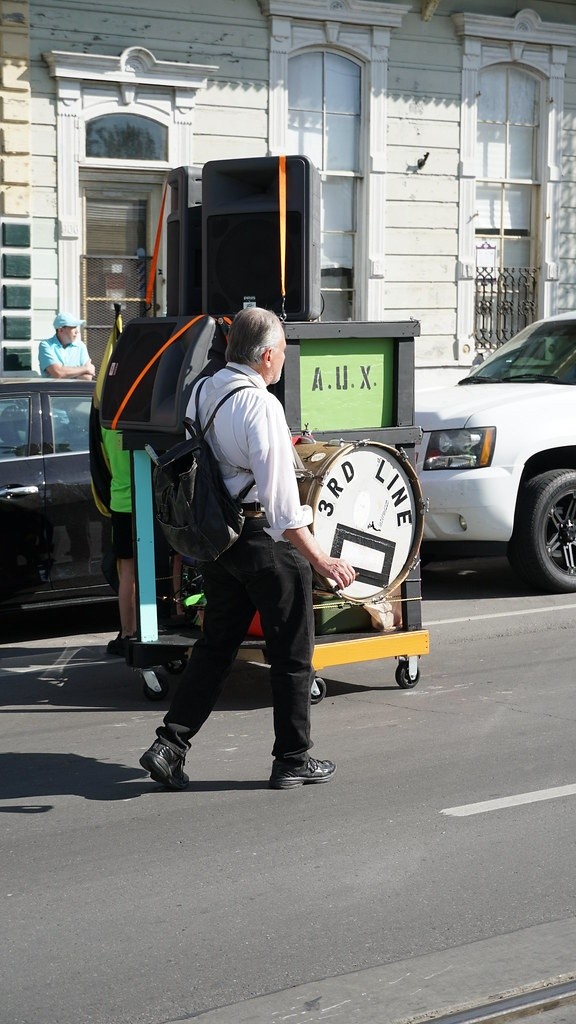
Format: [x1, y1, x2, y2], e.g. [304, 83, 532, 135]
[291, 422, 430, 609]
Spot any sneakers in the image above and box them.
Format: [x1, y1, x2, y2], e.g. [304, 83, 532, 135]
[139, 741, 190, 788]
[268, 757, 337, 789]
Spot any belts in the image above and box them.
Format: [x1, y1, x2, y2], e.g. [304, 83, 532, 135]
[242, 502, 264, 511]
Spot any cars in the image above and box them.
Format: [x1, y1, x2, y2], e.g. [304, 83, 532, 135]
[0, 372, 171, 643]
[415, 308, 574, 593]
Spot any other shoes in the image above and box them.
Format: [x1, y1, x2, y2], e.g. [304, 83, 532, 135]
[107, 632, 138, 657]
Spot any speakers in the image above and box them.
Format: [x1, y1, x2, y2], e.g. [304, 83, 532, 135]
[101, 154, 321, 434]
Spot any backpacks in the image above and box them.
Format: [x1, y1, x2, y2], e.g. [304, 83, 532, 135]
[152, 377, 256, 562]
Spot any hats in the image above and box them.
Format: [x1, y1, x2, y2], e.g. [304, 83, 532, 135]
[54, 313, 85, 329]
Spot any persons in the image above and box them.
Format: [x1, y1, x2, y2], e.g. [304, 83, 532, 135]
[102, 427, 137, 654]
[139, 308, 356, 788]
[39, 312, 94, 445]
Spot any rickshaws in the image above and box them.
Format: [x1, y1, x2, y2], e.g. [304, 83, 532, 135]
[88, 302, 432, 705]
